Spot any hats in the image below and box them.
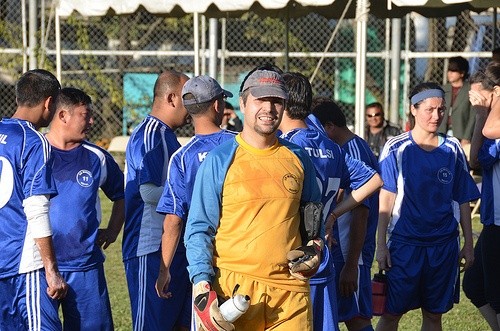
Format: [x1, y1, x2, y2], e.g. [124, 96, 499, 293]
[240, 70, 291, 99]
[181, 75, 233, 105]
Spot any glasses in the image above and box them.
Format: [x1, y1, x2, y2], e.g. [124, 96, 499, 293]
[448, 67, 458, 72]
[367, 111, 382, 117]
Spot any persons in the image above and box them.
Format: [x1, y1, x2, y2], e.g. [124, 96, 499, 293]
[373, 81, 482, 331]
[41, 86, 125, 331]
[436, 55, 477, 146]
[154, 74, 244, 331]
[462, 59, 500, 331]
[0, 69, 69, 331]
[121, 70, 193, 331]
[275, 71, 403, 331]
[184, 63, 327, 331]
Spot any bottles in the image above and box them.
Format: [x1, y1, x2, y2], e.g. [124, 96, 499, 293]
[217, 294, 251, 324]
[371, 268, 388, 316]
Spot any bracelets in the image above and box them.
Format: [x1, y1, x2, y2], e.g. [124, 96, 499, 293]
[330, 213, 336, 220]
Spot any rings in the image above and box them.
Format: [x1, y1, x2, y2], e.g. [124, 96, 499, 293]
[475, 99, 477, 101]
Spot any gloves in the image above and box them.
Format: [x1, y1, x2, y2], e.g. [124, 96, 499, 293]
[287, 236, 324, 282]
[192, 281, 236, 331]
